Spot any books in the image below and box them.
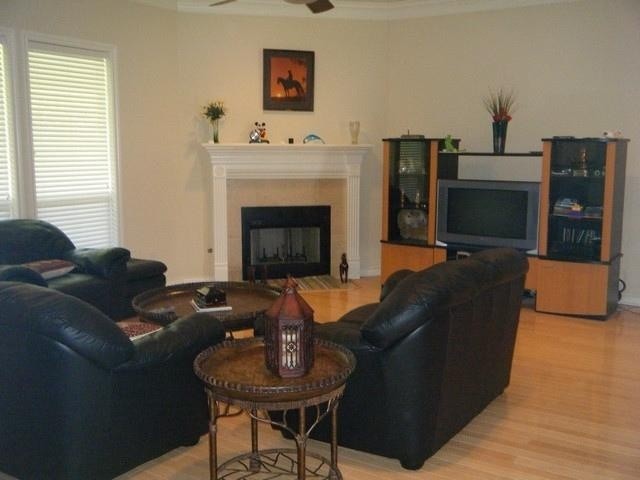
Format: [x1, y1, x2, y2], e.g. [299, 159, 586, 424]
[191, 296, 233, 313]
[561, 225, 602, 246]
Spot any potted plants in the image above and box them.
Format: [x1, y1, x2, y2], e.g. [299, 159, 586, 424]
[201, 99, 227, 143]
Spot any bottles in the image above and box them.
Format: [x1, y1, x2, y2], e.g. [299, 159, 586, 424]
[400, 188, 407, 208]
[415, 191, 421, 208]
[339, 254, 349, 283]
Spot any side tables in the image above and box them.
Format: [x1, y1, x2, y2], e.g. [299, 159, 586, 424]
[194, 334, 355, 480]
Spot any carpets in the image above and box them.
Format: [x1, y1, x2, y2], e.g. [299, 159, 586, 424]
[268, 274, 363, 291]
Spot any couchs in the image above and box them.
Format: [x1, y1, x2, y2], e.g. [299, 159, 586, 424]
[0, 219, 169, 321]
[0, 280, 225, 480]
[254, 246, 529, 471]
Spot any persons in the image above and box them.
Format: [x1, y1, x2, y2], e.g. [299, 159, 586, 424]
[285, 70, 293, 80]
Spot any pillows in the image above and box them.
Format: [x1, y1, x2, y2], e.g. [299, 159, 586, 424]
[24, 259, 75, 280]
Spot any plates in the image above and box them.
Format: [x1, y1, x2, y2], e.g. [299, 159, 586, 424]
[396, 208, 427, 229]
[399, 141, 425, 176]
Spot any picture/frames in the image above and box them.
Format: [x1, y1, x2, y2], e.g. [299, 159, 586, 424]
[262, 47, 314, 112]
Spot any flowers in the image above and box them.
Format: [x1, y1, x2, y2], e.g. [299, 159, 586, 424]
[482, 89, 516, 120]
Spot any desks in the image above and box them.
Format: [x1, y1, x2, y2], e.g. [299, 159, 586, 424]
[131, 281, 283, 330]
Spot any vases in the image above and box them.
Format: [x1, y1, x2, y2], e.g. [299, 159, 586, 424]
[493, 122, 507, 152]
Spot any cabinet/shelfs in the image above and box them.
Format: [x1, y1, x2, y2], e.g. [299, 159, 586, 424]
[379, 135, 632, 320]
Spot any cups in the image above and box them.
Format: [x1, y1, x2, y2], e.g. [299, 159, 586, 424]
[349, 120, 360, 145]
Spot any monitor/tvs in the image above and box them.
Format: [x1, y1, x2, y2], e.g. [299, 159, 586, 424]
[436, 178, 539, 252]
